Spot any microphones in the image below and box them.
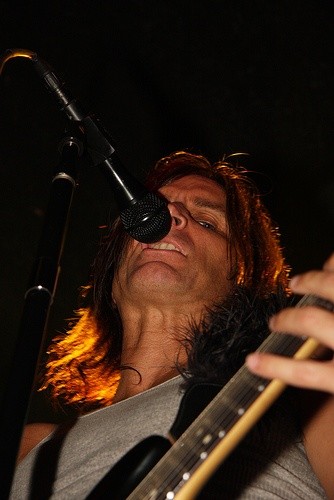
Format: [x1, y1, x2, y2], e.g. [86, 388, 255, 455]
[34, 60, 173, 243]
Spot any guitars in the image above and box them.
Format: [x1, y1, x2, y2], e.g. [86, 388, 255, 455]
[121, 256, 333, 500]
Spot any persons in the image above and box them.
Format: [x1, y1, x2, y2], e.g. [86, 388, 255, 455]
[7, 150, 334, 500]
